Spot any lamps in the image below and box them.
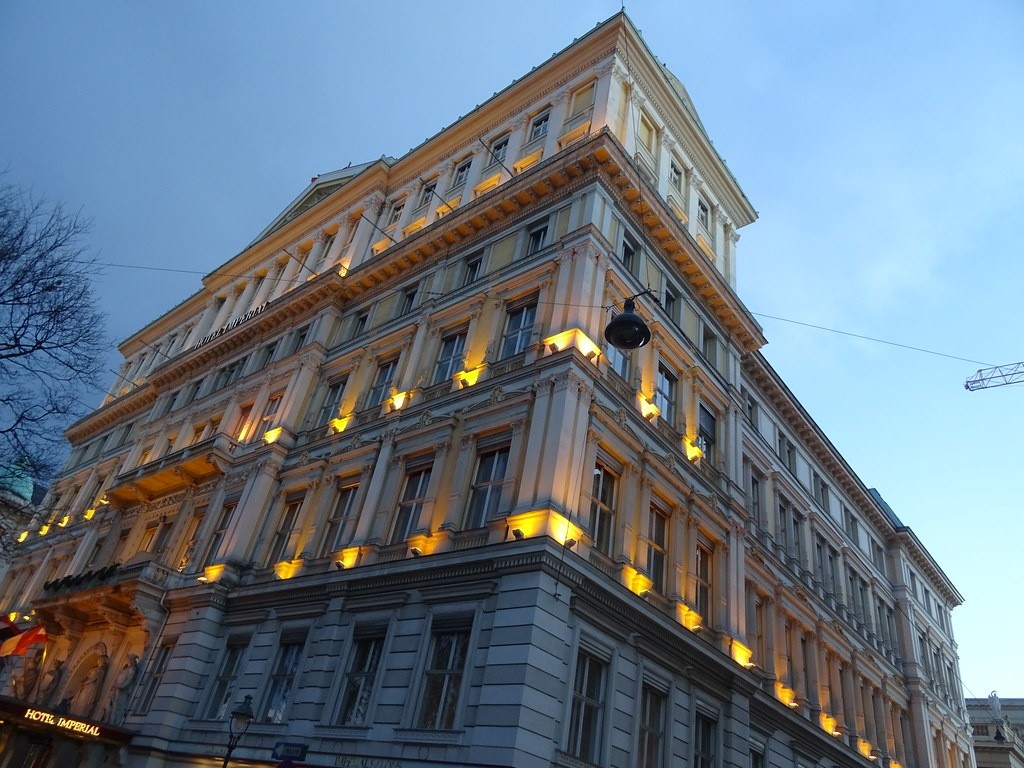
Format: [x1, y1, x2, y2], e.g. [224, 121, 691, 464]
[411, 547, 422, 557]
[335, 560, 346, 570]
[585, 351, 596, 359]
[549, 343, 559, 354]
[789, 702, 800, 709]
[744, 663, 755, 670]
[690, 455, 699, 462]
[869, 756, 878, 762]
[692, 626, 703, 635]
[332, 426, 340, 434]
[833, 732, 842, 737]
[640, 590, 651, 598]
[389, 402, 398, 411]
[602, 299, 652, 350]
[565, 538, 576, 550]
[262, 437, 269, 445]
[645, 411, 655, 421]
[511, 529, 523, 540]
[197, 577, 209, 584]
[272, 573, 282, 579]
[460, 379, 470, 387]
[993, 726, 1006, 744]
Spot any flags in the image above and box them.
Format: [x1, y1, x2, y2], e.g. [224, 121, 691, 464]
[0, 626, 47, 656]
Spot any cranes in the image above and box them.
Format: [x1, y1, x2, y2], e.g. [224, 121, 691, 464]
[963, 361, 1024, 392]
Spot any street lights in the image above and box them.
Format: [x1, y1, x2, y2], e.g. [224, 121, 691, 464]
[222, 695, 254, 768]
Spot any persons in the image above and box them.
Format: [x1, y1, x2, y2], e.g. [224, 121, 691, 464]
[991, 693, 1001, 718]
[34, 660, 63, 707]
[11, 659, 39, 699]
[69, 655, 107, 718]
[96, 654, 139, 726]
